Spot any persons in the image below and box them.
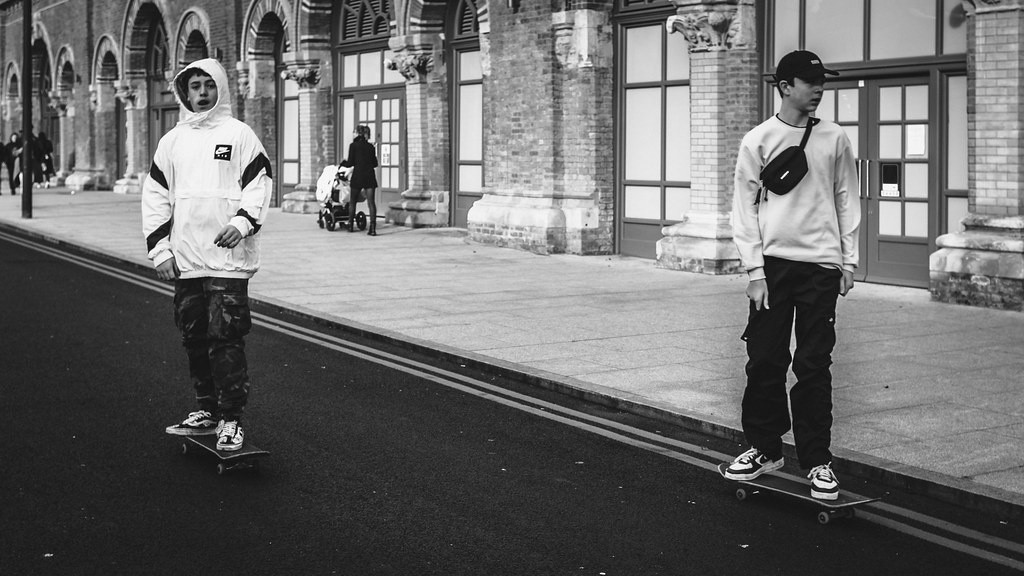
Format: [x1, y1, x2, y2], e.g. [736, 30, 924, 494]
[19, 124, 57, 188]
[141, 57, 273, 453]
[719, 49, 862, 502]
[0, 141, 5, 194]
[340, 124, 378, 235]
[4, 133, 23, 195]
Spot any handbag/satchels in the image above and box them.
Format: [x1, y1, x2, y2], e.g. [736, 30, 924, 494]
[756, 145, 808, 195]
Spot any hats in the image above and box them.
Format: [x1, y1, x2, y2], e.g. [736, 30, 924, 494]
[776, 50, 839, 80]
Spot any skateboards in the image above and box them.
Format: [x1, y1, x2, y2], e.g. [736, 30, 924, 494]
[716, 462, 884, 525]
[180, 424, 271, 476]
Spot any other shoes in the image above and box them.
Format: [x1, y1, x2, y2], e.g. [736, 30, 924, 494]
[341, 221, 354, 232]
[367, 222, 376, 235]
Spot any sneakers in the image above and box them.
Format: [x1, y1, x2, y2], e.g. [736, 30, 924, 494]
[811, 462, 840, 501]
[165, 410, 218, 435]
[216, 419, 245, 451]
[724, 438, 784, 481]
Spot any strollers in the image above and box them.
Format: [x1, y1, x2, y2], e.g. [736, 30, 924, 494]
[316, 161, 367, 232]
